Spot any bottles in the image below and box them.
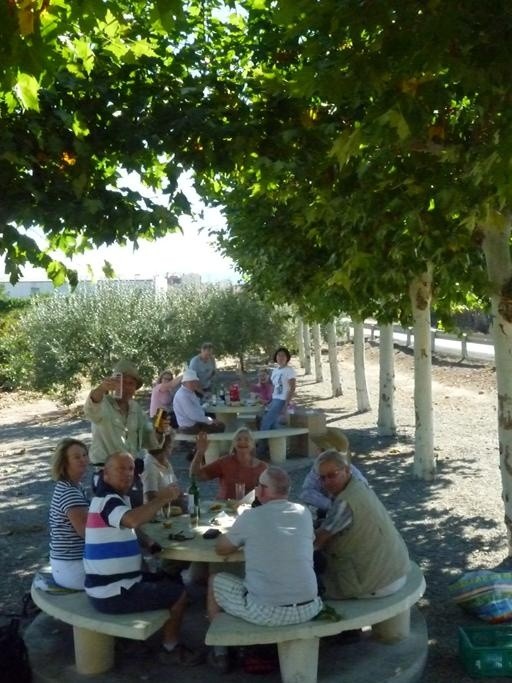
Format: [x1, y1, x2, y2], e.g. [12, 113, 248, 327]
[211, 383, 226, 407]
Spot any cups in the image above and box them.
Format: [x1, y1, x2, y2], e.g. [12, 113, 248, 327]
[108, 370, 124, 402]
[159, 480, 249, 530]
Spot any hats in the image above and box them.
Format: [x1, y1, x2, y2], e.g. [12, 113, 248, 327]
[113, 360, 143, 388]
[181, 369, 199, 383]
[310, 427, 350, 454]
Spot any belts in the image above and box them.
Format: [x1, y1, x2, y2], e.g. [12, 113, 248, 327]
[280, 599, 315, 608]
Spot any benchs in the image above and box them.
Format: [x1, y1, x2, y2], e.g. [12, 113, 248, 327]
[29, 565, 172, 675]
[204, 559, 426, 683]
[236, 407, 326, 458]
[173, 427, 309, 466]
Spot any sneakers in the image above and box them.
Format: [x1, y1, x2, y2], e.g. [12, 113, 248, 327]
[207, 650, 231, 674]
[337, 630, 360, 643]
[187, 451, 194, 462]
[158, 642, 203, 665]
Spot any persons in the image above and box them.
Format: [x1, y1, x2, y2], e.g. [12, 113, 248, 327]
[205, 464, 326, 674]
[257, 347, 296, 429]
[83, 449, 200, 669]
[311, 451, 411, 645]
[83, 358, 173, 510]
[189, 342, 217, 400]
[188, 424, 270, 502]
[298, 424, 369, 575]
[142, 424, 195, 589]
[232, 362, 273, 402]
[171, 368, 227, 461]
[47, 435, 97, 591]
[148, 369, 188, 444]
